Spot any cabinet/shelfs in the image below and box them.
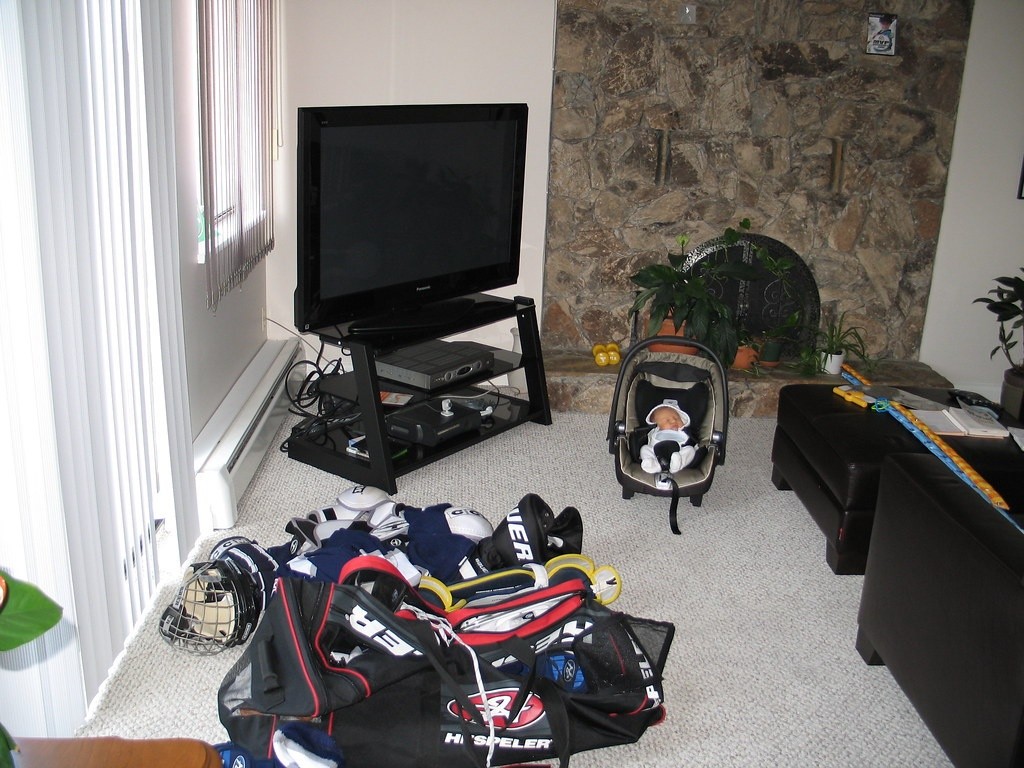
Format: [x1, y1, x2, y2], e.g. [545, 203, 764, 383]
[286, 291, 553, 496]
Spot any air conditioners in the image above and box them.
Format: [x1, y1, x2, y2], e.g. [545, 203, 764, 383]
[195, 336, 308, 529]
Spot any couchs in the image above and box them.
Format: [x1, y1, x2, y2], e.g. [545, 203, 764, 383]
[856, 451, 1024, 768]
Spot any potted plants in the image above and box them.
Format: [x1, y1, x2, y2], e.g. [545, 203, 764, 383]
[625, 217, 879, 379]
[972, 267, 1024, 416]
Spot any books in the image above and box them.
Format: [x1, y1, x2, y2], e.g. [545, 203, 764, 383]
[908, 396, 1009, 439]
[1007, 426, 1024, 451]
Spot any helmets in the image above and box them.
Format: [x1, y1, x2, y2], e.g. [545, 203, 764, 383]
[157, 560, 262, 658]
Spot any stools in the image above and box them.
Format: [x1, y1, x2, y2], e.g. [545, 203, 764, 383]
[772, 382, 1024, 576]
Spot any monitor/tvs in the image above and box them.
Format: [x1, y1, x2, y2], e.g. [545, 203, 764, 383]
[294, 102, 528, 333]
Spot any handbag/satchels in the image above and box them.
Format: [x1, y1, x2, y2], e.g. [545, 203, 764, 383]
[217, 576, 675, 768]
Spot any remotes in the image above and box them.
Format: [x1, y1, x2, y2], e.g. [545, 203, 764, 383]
[947, 387, 1006, 414]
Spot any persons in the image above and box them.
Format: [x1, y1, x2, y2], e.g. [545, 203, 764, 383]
[639, 403, 696, 473]
[879, 17, 894, 39]
